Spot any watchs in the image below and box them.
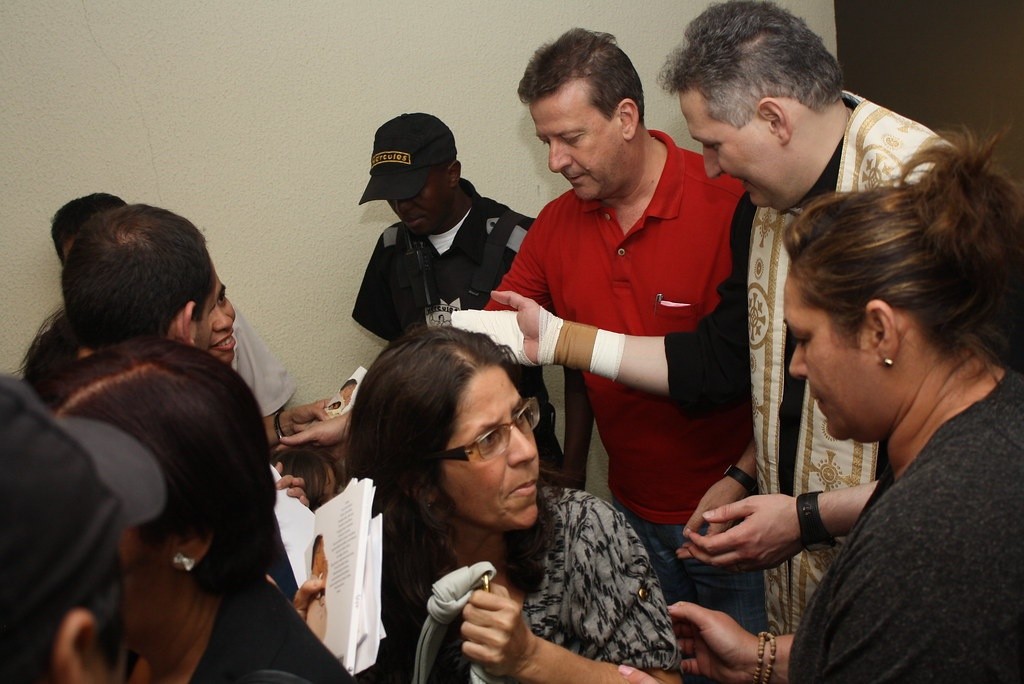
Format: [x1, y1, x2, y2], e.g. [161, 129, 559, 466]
[797, 491, 837, 552]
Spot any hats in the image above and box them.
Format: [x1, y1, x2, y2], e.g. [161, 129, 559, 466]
[358, 114, 458, 205]
[0, 368, 168, 666]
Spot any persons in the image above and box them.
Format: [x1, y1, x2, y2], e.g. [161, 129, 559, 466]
[307, 535, 327, 643]
[347, 326, 682, 684]
[451, 0, 954, 635]
[26, 340, 356, 684]
[0, 374, 164, 684]
[351, 112, 594, 489]
[485, 29, 769, 684]
[53, 194, 341, 587]
[325, 379, 357, 417]
[619, 144, 1024, 684]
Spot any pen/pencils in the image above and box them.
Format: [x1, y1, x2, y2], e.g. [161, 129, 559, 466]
[654, 292, 663, 317]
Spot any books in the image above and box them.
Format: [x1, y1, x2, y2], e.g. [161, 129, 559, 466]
[324, 365, 367, 418]
[270, 465, 382, 676]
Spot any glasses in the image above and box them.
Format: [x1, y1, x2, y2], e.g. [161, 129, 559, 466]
[413, 394, 540, 463]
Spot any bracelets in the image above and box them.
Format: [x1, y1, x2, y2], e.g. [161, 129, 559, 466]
[724, 464, 759, 496]
[754, 632, 776, 684]
[275, 409, 285, 440]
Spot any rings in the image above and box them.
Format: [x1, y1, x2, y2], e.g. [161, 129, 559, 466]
[736, 564, 747, 576]
